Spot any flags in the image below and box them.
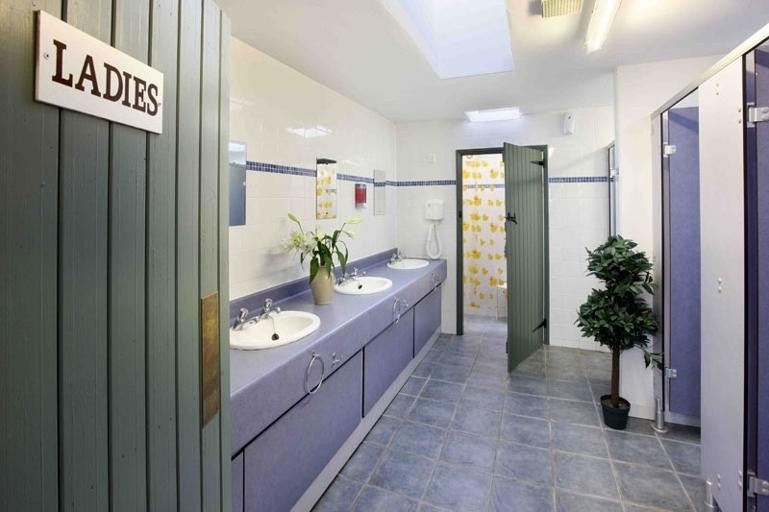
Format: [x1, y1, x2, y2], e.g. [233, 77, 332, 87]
[310, 266, 335, 305]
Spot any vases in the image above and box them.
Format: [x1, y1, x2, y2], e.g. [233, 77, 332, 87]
[310, 266, 335, 305]
[282, 212, 363, 285]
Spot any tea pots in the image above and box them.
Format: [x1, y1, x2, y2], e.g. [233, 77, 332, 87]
[316, 156, 337, 219]
[374, 169, 387, 216]
[230, 141, 247, 225]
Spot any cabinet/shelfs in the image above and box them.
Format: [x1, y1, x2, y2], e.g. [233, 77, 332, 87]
[231, 283, 442, 512]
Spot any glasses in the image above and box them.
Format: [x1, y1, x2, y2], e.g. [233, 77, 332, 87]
[582, 0, 622, 52]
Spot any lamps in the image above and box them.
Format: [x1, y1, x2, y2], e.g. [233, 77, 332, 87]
[582, 0, 622, 52]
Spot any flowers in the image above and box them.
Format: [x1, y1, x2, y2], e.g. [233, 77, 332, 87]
[282, 212, 363, 285]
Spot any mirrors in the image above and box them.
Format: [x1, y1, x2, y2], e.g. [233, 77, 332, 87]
[316, 156, 337, 219]
[374, 169, 387, 216]
[230, 141, 247, 225]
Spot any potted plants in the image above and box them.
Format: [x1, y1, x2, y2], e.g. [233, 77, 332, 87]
[573, 235, 667, 429]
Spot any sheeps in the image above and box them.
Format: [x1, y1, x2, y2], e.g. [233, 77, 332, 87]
[338, 272, 356, 286]
[391, 251, 403, 263]
[234, 308, 261, 330]
[261, 298, 281, 318]
[350, 267, 367, 277]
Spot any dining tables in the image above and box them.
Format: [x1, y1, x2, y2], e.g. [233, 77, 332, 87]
[573, 235, 667, 429]
